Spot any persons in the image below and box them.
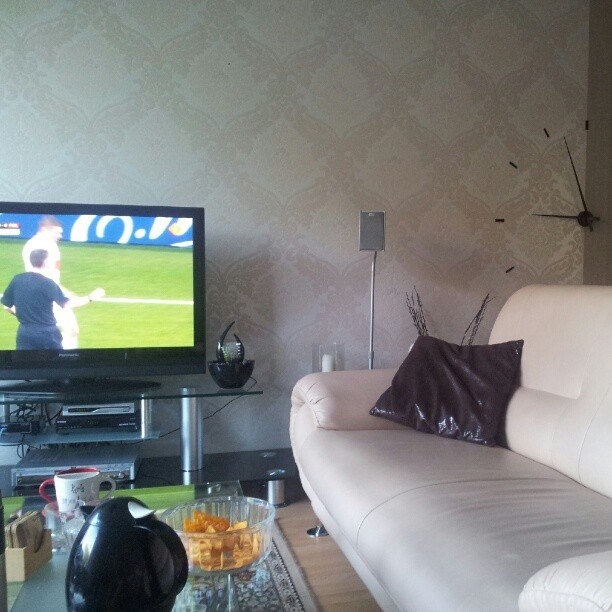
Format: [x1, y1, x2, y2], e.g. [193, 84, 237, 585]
[0, 250, 106, 349]
[22, 215, 80, 350]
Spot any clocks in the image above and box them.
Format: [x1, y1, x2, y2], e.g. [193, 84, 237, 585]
[493, 118, 612, 287]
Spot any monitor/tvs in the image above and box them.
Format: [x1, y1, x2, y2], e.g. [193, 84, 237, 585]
[0, 201, 207, 400]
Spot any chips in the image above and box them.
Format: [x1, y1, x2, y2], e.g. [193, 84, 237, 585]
[182, 509, 262, 570]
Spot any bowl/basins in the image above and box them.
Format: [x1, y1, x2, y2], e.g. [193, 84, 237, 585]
[162, 493, 276, 583]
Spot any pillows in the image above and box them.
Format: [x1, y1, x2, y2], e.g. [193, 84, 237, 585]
[369, 335, 523, 447]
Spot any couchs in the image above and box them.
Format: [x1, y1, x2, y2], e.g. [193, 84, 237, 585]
[289, 284, 611, 612]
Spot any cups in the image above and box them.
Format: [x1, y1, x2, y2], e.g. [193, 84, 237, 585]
[44, 500, 83, 552]
[41, 472, 114, 515]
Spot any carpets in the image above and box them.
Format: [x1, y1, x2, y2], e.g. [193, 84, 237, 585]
[172, 519, 324, 612]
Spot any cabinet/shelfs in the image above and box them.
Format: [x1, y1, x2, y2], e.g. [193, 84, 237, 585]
[0, 366, 298, 507]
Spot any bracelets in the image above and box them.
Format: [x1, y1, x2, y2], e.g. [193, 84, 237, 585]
[87, 295, 93, 303]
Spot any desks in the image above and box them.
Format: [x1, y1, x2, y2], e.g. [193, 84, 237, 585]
[8, 480, 285, 611]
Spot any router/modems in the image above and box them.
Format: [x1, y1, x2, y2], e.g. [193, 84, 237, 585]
[1, 414, 47, 436]
